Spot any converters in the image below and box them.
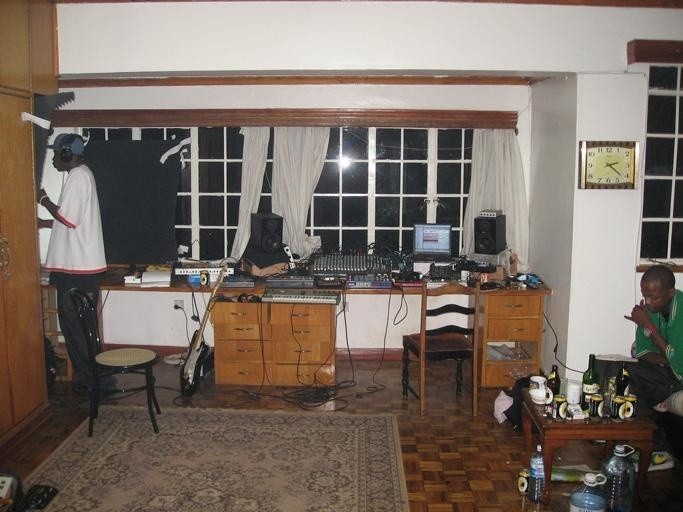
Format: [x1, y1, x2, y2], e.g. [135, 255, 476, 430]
[316, 275, 342, 288]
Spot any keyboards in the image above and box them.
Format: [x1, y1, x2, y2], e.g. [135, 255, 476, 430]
[429, 266, 453, 278]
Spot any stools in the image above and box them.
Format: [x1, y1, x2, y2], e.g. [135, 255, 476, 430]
[85, 347, 161, 437]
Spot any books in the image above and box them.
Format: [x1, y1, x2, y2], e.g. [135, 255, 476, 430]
[141, 271, 171, 282]
[139, 281, 170, 288]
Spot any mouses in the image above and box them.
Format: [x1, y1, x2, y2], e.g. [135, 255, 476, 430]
[481, 282, 499, 290]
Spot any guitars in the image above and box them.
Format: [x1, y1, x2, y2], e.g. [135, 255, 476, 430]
[180, 264, 228, 395]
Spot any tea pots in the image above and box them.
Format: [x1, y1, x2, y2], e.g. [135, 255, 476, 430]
[529, 375, 553, 405]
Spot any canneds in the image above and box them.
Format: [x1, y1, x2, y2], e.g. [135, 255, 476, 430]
[590, 393, 604, 416]
[611, 395, 625, 420]
[517, 469, 531, 495]
[200, 271, 210, 287]
[553, 394, 566, 422]
[625, 394, 637, 417]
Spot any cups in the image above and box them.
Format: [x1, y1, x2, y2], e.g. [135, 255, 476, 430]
[565, 384, 581, 404]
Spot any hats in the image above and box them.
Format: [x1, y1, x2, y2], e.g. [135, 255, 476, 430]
[46, 133, 84, 152]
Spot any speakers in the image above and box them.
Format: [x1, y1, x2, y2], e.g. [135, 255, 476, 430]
[251, 212, 283, 254]
[474, 215, 506, 255]
[593, 359, 638, 388]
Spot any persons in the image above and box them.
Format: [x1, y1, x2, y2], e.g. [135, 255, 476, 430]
[623, 265, 683, 473]
[35, 133, 107, 398]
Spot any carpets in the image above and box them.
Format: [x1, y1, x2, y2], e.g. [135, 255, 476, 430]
[20, 401, 413, 512]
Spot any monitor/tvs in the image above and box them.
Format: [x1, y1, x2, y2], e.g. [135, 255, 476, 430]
[413, 223, 452, 261]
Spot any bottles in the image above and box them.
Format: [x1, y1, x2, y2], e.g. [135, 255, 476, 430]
[581, 353, 600, 406]
[529, 444, 546, 503]
[616, 361, 633, 395]
[600, 444, 636, 512]
[546, 364, 561, 395]
[569, 472, 610, 512]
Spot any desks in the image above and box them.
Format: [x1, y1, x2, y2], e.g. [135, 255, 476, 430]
[517, 385, 659, 506]
[41, 283, 209, 383]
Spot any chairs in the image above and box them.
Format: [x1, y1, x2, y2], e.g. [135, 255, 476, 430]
[401, 281, 482, 417]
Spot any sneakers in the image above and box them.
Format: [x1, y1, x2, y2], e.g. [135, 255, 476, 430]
[628, 451, 674, 472]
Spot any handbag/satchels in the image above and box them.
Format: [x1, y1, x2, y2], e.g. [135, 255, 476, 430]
[510, 374, 548, 433]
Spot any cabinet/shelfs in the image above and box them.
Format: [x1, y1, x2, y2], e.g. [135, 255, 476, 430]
[213, 281, 336, 389]
[0, 0, 59, 461]
[466, 289, 544, 389]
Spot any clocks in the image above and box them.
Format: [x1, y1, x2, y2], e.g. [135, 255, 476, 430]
[577, 139, 640, 191]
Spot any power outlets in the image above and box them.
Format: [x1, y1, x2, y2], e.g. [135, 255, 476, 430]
[173, 299, 185, 311]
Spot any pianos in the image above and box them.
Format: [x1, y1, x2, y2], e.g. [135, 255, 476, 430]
[262, 288, 340, 305]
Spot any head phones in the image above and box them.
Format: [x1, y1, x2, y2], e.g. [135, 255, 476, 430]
[238, 292, 260, 303]
[60, 134, 84, 162]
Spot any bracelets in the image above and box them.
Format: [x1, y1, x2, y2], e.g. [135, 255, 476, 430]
[643, 326, 656, 337]
[37, 195, 49, 204]
